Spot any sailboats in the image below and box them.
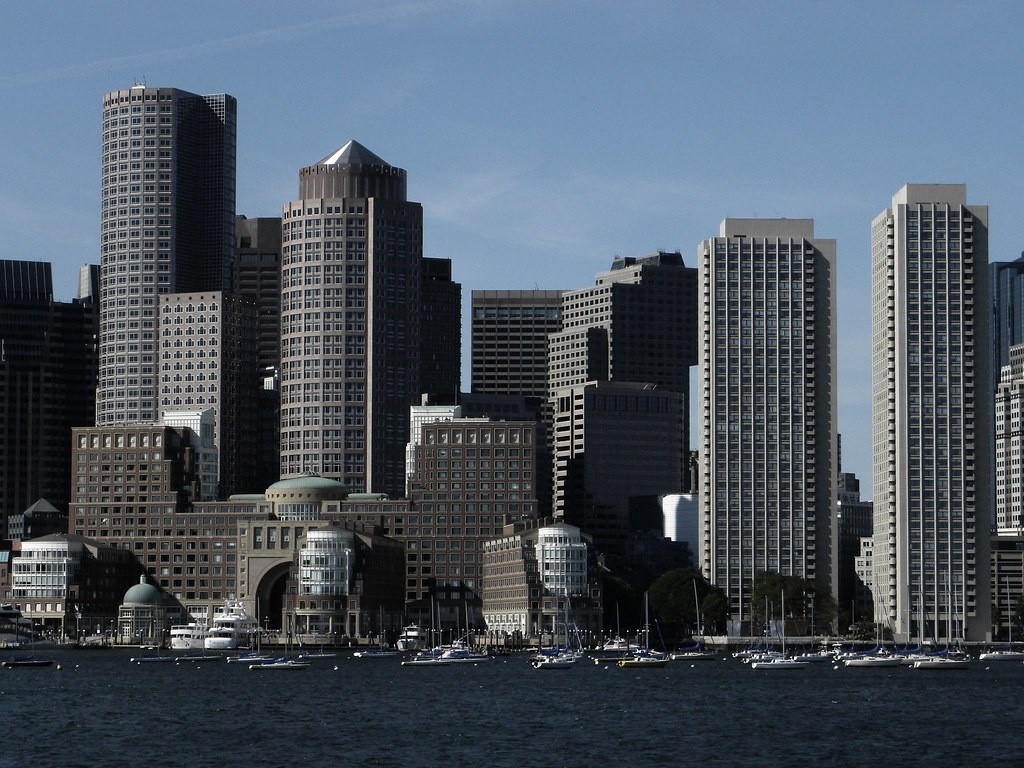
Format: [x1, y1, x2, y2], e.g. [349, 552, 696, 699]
[1, 568, 1023, 670]
